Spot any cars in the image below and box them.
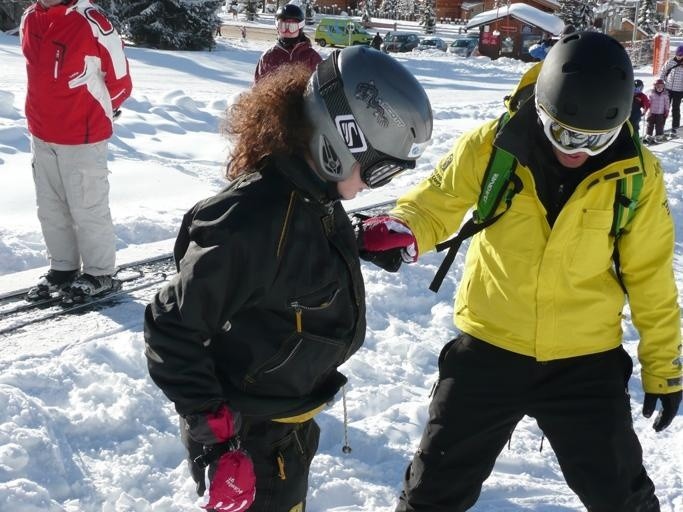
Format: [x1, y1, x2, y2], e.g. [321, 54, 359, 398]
[444, 39, 478, 58]
[418, 37, 447, 53]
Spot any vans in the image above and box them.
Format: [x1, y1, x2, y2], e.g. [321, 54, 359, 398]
[314, 17, 372, 47]
[379, 31, 420, 53]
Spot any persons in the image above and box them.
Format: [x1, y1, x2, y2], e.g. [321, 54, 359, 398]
[215, 22, 222, 36]
[642, 78, 670, 146]
[657, 46, 683, 142]
[141, 47, 435, 512]
[253, 4, 325, 87]
[355, 31, 682, 512]
[627, 79, 651, 138]
[239, 25, 247, 39]
[18, 0, 136, 308]
[370, 30, 384, 51]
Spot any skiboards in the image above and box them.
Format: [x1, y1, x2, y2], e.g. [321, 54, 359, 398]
[1, 270, 165, 335]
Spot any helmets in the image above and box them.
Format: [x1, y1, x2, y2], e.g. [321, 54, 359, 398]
[274, 3, 305, 32]
[676, 46, 683, 56]
[653, 79, 665, 88]
[534, 30, 636, 134]
[285, 45, 435, 184]
[362, 158, 417, 190]
[634, 79, 644, 91]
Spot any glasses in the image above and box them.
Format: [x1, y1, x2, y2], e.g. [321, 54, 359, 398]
[278, 21, 299, 34]
[543, 117, 623, 157]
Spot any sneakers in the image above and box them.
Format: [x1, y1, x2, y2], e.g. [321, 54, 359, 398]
[642, 127, 680, 144]
[24, 268, 82, 302]
[58, 273, 114, 308]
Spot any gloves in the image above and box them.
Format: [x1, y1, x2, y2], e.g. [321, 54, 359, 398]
[642, 390, 683, 433]
[184, 405, 258, 512]
[664, 110, 669, 117]
[350, 213, 419, 273]
